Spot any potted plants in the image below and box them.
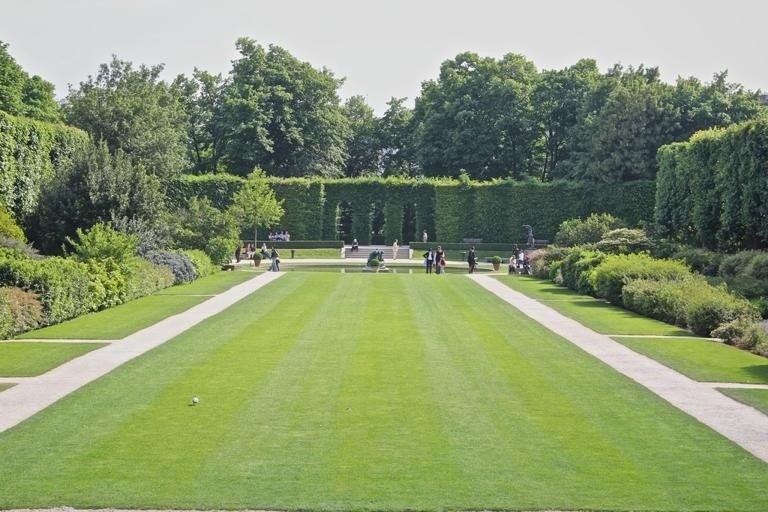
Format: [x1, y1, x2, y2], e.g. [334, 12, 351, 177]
[253, 253, 262, 267]
[492, 256, 502, 270]
[370, 259, 379, 272]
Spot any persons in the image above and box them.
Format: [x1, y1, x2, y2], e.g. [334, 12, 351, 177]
[423, 247, 434, 274]
[349, 238, 359, 253]
[232, 230, 291, 272]
[466, 246, 476, 274]
[433, 245, 445, 274]
[438, 255, 447, 274]
[421, 229, 428, 242]
[507, 244, 532, 276]
[391, 239, 400, 260]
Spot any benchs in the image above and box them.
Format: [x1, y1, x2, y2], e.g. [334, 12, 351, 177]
[509, 268, 525, 275]
[243, 253, 265, 261]
[223, 263, 241, 271]
